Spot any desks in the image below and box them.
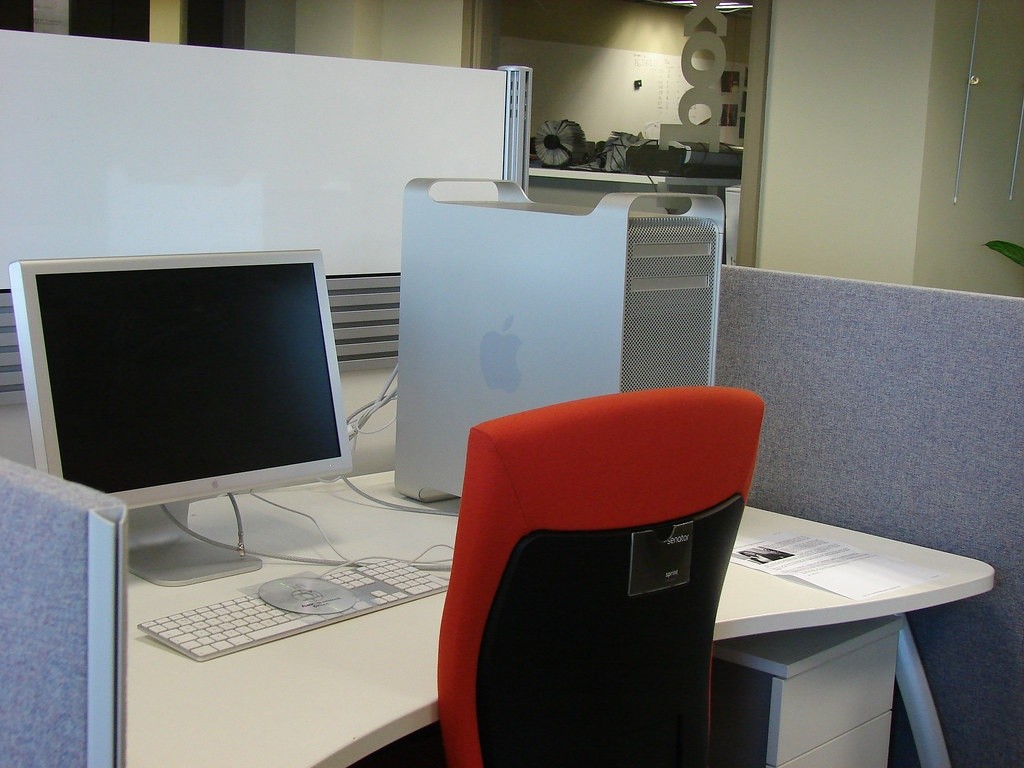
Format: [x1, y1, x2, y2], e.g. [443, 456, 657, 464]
[0, 476, 996, 768]
[527, 167, 742, 214]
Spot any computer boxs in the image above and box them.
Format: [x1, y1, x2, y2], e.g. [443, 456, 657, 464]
[394, 180, 727, 503]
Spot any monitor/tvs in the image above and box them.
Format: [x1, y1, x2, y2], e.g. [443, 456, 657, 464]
[9, 249, 355, 585]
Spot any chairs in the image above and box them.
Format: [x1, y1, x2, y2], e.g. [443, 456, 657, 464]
[438, 385, 765, 768]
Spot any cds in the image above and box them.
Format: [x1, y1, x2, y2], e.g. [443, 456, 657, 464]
[261, 577, 355, 614]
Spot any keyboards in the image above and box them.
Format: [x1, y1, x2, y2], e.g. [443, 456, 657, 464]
[134, 559, 449, 661]
